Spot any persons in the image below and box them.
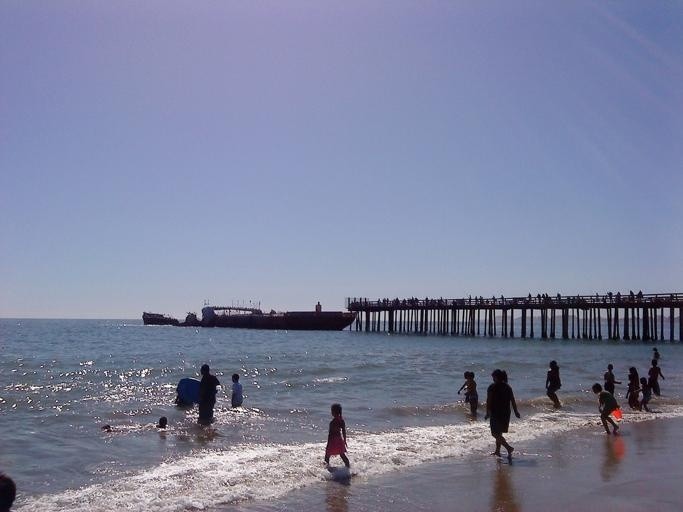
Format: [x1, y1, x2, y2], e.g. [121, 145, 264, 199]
[197, 364, 220, 423]
[231, 374, 243, 408]
[349, 290, 644, 307]
[0, 470, 17, 512]
[102, 423, 114, 433]
[484, 369, 520, 457]
[592, 347, 666, 435]
[324, 404, 349, 468]
[545, 360, 562, 408]
[458, 371, 478, 416]
[155, 416, 169, 430]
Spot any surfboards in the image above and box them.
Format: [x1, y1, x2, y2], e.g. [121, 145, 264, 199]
[178, 379, 202, 405]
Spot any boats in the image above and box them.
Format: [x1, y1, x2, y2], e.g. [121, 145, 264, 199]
[202, 296, 359, 328]
[174, 313, 201, 326]
[141, 313, 178, 326]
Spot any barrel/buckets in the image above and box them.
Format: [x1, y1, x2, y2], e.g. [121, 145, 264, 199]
[612, 408, 625, 421]
